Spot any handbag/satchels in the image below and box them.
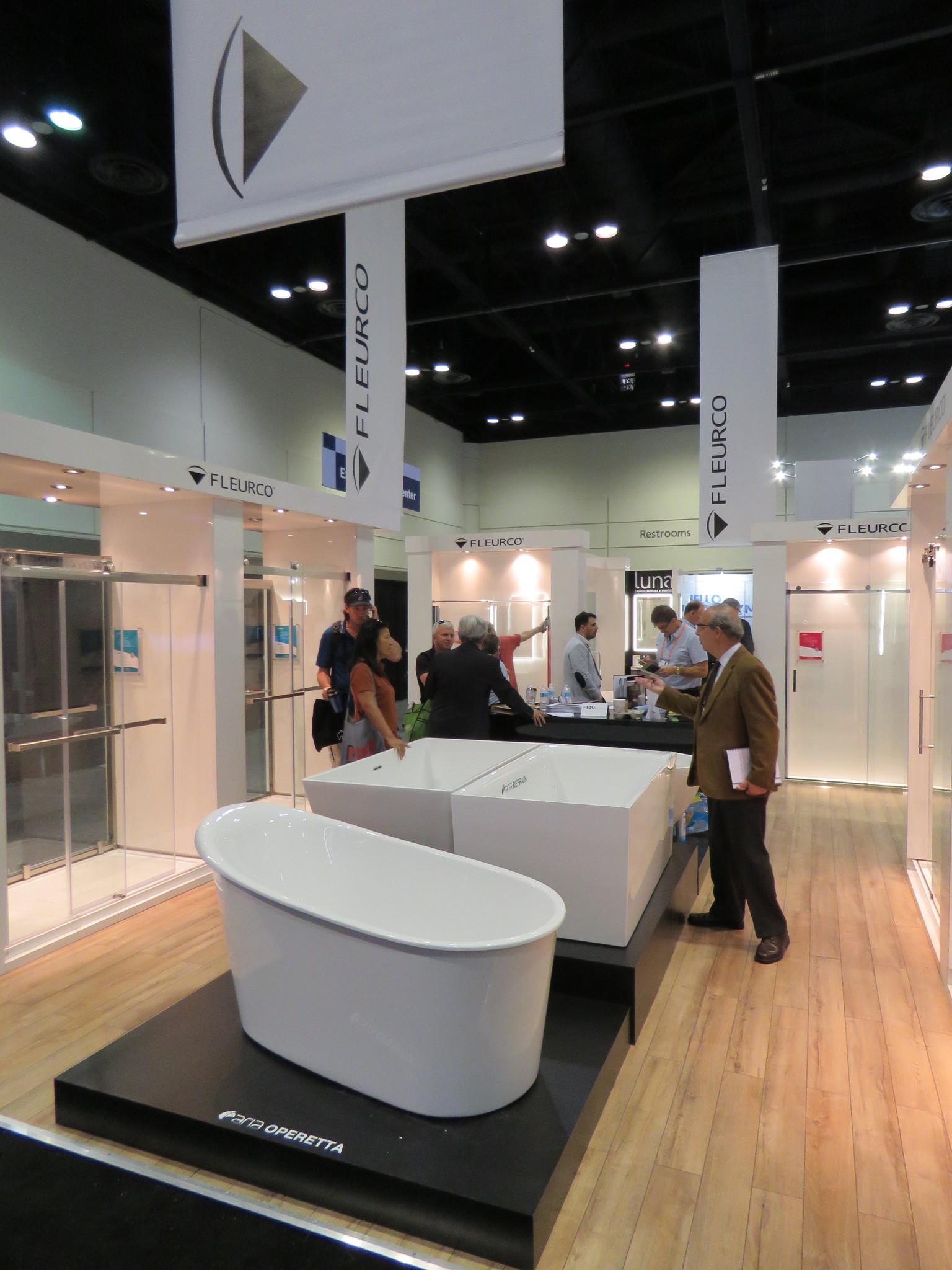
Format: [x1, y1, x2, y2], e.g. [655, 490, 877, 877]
[403, 699, 434, 743]
[339, 670, 387, 766]
[311, 699, 352, 753]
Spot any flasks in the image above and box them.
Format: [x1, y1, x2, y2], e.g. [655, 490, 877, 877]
[327, 687, 345, 713]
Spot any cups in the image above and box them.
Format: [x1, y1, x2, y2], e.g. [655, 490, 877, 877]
[614, 699, 628, 719]
[532, 688, 537, 705]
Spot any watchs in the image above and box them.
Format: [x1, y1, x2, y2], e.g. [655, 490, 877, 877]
[675, 667, 681, 675]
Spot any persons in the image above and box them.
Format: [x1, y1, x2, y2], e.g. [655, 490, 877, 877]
[422, 614, 551, 740]
[415, 619, 456, 705]
[679, 600, 705, 630]
[708, 597, 755, 678]
[479, 633, 511, 709]
[338, 618, 411, 766]
[316, 586, 402, 769]
[560, 611, 607, 707]
[633, 603, 792, 965]
[484, 618, 549, 693]
[637, 604, 710, 698]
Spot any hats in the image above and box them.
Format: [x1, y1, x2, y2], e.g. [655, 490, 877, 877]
[344, 588, 372, 607]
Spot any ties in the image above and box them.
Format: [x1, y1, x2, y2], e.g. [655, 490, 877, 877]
[703, 660, 721, 708]
[589, 648, 602, 680]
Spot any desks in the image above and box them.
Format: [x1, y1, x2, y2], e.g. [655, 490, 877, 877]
[498, 704, 697, 754]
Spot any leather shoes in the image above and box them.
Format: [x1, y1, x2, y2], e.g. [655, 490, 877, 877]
[754, 929, 790, 963]
[688, 912, 745, 929]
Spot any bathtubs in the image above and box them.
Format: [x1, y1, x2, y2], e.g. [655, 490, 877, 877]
[194, 803, 569, 1119]
[451, 744, 679, 948]
[301, 736, 543, 855]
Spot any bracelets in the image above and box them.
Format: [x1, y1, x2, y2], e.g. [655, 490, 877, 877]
[536, 626, 540, 632]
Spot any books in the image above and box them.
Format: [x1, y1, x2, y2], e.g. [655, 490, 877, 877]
[725, 747, 782, 790]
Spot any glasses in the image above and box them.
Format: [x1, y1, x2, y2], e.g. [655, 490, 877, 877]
[344, 589, 371, 600]
[438, 620, 451, 624]
[697, 621, 719, 629]
[738, 610, 742, 616]
[654, 616, 675, 630]
[376, 618, 382, 624]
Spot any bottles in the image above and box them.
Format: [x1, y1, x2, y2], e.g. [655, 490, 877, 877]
[563, 684, 571, 704]
[540, 685, 548, 716]
[678, 813, 687, 843]
[526, 683, 534, 705]
[547, 683, 555, 705]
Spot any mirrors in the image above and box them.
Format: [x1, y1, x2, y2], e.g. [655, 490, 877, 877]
[677, 568, 753, 635]
[632, 593, 673, 651]
